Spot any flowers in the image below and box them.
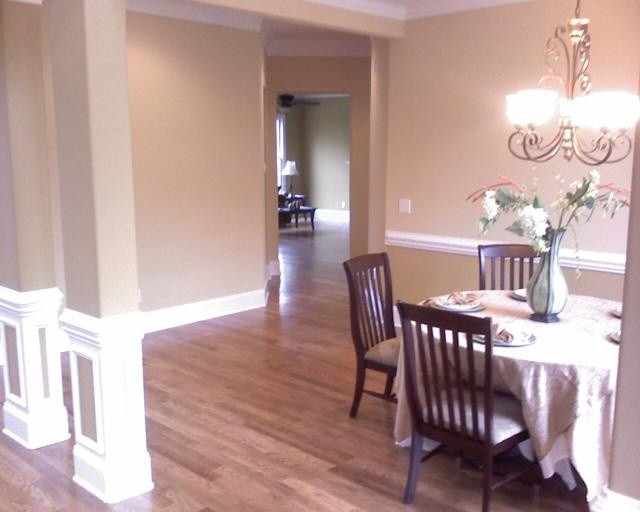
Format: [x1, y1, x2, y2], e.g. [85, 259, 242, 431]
[465, 168, 631, 257]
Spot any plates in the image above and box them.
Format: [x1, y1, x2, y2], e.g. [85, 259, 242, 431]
[432, 288, 623, 348]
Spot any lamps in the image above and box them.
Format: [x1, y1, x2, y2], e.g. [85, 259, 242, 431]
[281, 159, 300, 197]
[504, 1, 640, 165]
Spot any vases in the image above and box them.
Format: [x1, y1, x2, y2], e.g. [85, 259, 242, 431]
[525, 226, 571, 322]
